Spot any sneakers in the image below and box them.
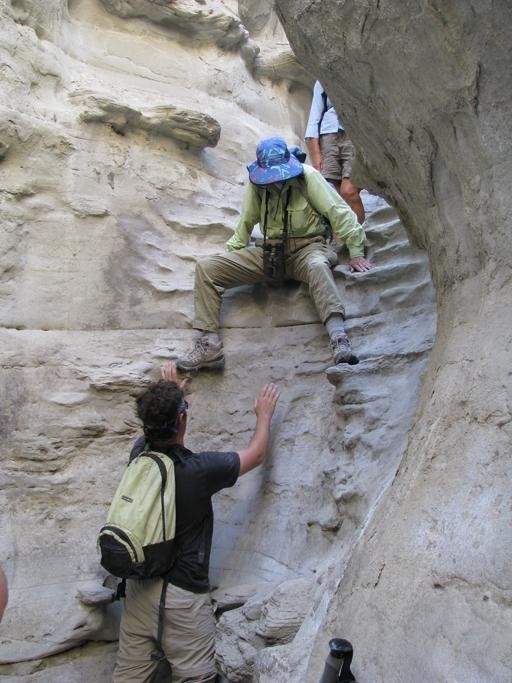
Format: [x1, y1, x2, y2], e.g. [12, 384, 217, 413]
[326, 332, 360, 365]
[175, 335, 225, 372]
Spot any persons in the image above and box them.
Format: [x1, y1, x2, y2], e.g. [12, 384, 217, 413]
[103, 359, 281, 682]
[304, 80, 368, 247]
[174, 135, 368, 373]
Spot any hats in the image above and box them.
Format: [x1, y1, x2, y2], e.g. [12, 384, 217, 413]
[245, 136, 304, 186]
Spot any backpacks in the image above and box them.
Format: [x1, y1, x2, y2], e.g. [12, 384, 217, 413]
[97, 440, 192, 582]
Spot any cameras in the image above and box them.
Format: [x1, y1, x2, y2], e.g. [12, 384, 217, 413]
[263, 242, 285, 280]
